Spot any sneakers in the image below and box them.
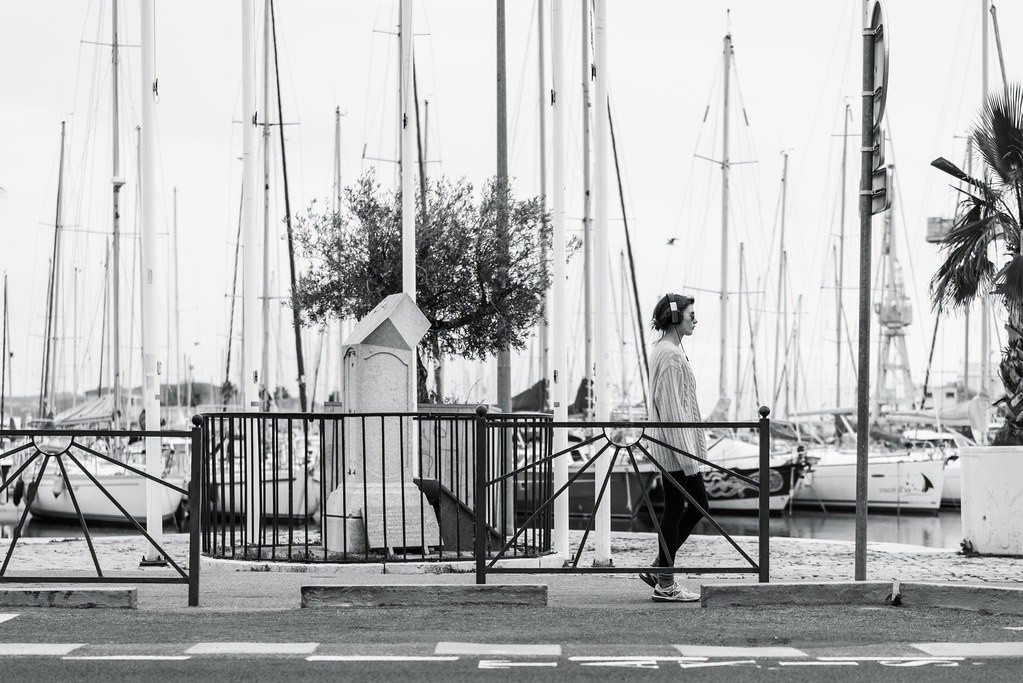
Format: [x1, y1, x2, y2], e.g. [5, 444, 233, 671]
[639, 572, 658, 588]
[651, 581, 701, 602]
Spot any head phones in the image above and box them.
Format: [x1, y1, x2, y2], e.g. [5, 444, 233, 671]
[666, 293, 682, 325]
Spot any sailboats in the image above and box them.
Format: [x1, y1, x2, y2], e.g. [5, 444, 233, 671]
[0, 2, 1008, 524]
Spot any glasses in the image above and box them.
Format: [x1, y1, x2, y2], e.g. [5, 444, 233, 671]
[680, 312, 694, 320]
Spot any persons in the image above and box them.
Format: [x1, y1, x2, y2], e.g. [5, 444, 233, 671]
[640, 293, 710, 602]
